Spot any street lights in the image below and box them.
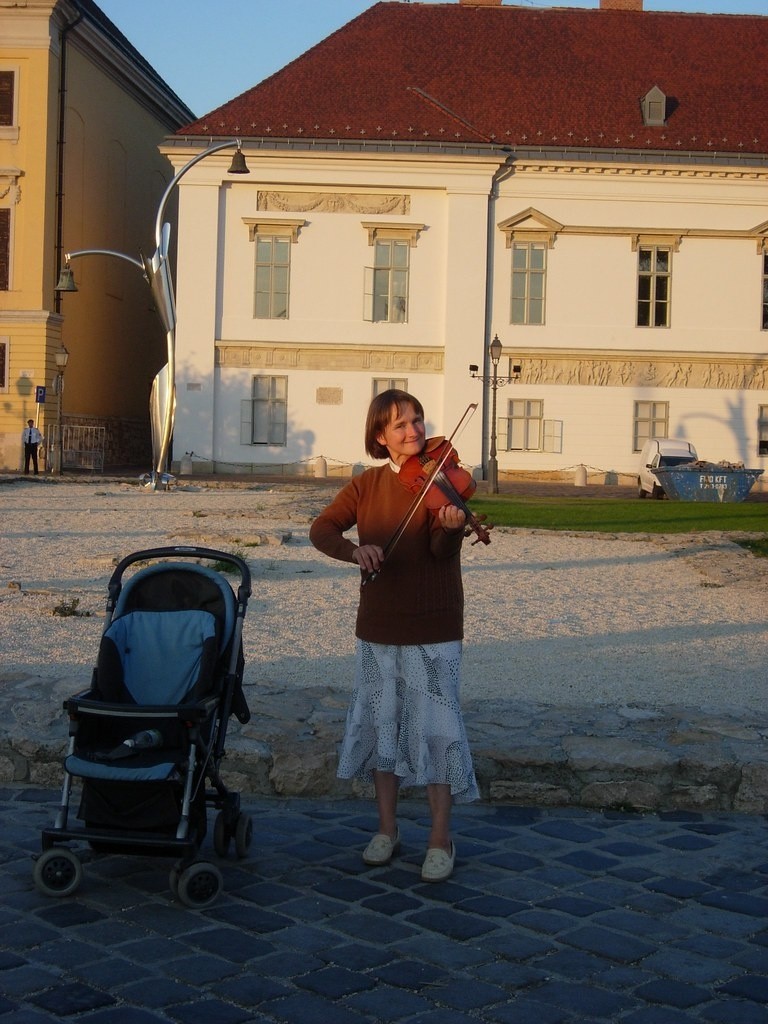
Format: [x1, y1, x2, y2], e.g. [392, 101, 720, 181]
[470, 337, 522, 495]
[53, 343, 71, 476]
[53, 136, 251, 490]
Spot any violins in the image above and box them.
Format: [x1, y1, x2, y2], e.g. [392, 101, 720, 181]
[396, 436, 495, 546]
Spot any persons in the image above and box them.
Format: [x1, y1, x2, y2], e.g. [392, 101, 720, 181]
[309, 388, 478, 881]
[23, 420, 42, 475]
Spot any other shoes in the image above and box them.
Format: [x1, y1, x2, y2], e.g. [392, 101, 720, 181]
[420, 839, 456, 882]
[362, 824, 401, 865]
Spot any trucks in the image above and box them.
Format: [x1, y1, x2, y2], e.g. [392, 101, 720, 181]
[636, 437, 698, 501]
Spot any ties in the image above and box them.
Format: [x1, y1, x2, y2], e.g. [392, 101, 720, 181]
[29, 429, 31, 443]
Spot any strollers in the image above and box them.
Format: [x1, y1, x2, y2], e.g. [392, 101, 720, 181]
[32, 544, 253, 910]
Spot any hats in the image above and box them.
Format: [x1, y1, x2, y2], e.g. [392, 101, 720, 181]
[28, 420, 33, 423]
